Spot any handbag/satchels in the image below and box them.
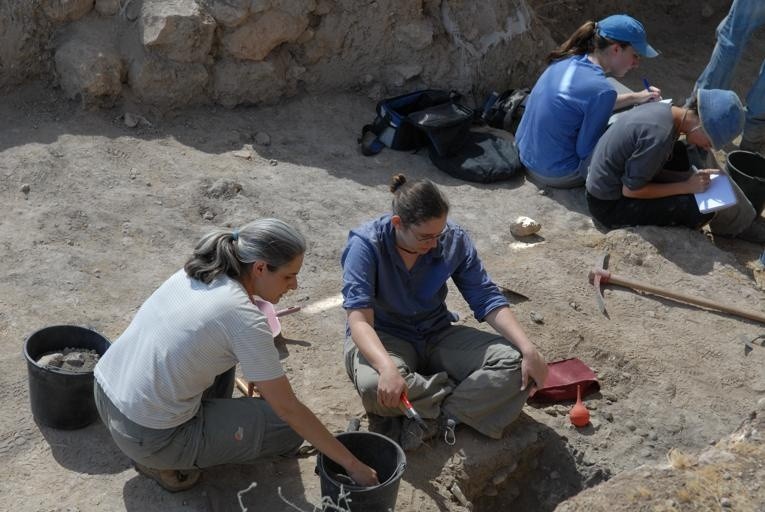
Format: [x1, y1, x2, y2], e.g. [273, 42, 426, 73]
[357, 87, 531, 183]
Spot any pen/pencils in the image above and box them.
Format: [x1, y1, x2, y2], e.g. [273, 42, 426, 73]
[643, 78, 649, 92]
[691, 165, 700, 175]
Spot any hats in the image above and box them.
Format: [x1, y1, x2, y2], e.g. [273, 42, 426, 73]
[697, 88, 745, 151]
[596, 13, 659, 58]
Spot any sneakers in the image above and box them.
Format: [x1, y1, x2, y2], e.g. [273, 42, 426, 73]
[130, 459, 201, 494]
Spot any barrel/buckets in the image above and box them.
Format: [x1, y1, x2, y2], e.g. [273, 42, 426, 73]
[316, 429, 407, 512]
[24, 325, 113, 431]
[724, 150, 765, 216]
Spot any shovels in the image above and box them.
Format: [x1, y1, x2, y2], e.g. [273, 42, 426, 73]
[738, 333, 765, 351]
[397, 393, 432, 451]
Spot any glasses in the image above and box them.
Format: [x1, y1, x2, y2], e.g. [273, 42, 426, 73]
[408, 223, 451, 241]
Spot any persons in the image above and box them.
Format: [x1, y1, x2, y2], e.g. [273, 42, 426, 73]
[585, 88, 747, 230]
[93, 216, 379, 492]
[684, 0, 764, 141]
[340, 174, 548, 450]
[516, 14, 660, 189]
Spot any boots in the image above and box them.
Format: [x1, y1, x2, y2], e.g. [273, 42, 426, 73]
[400, 419, 456, 451]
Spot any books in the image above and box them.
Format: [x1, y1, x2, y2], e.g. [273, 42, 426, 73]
[694, 174, 737, 213]
